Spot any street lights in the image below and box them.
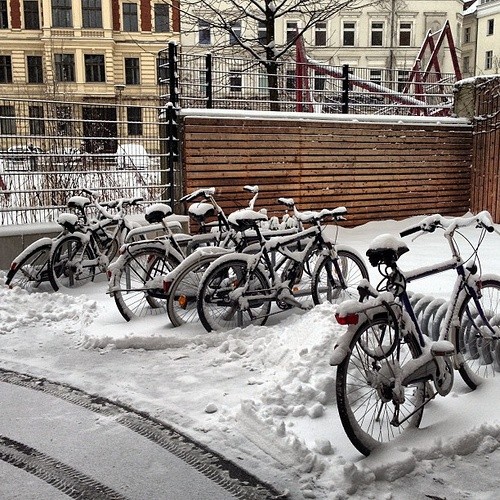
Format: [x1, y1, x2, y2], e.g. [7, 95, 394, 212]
[113, 84, 126, 145]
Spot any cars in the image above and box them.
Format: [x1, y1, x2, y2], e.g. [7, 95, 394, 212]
[7, 145, 46, 163]
[115, 144, 151, 170]
[47, 147, 93, 165]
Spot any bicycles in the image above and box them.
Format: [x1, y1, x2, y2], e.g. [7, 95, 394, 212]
[5, 190, 500, 456]
